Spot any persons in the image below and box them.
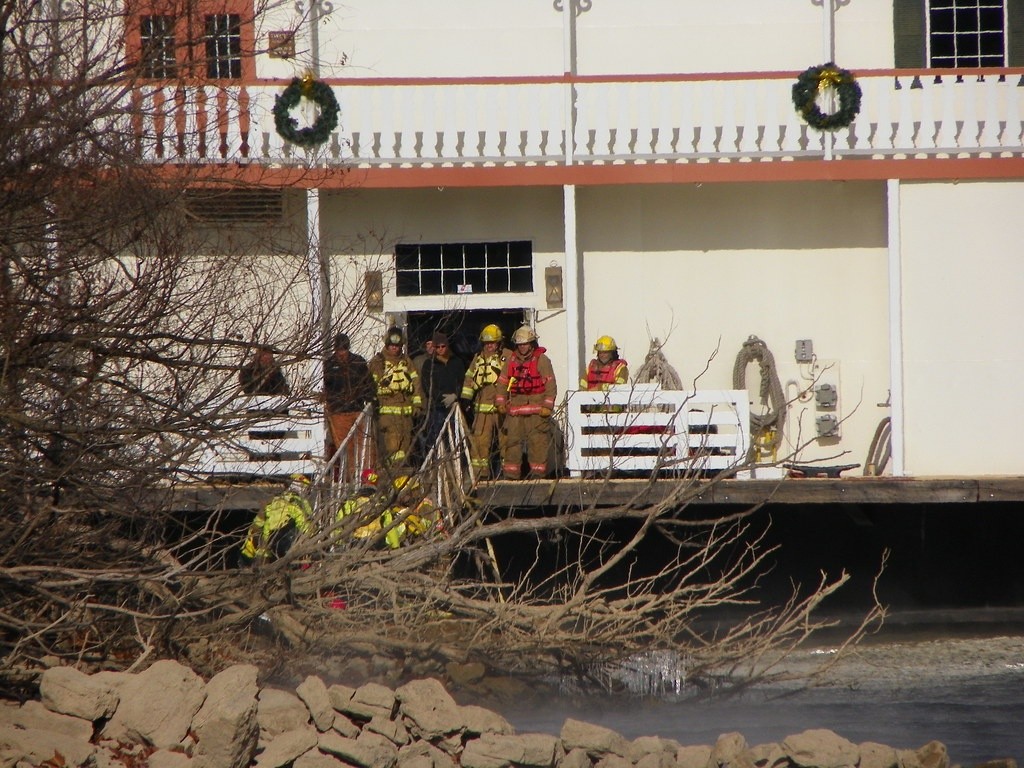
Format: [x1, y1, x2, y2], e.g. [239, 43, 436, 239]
[324, 331, 378, 482]
[457, 323, 514, 482]
[579, 335, 629, 478]
[238, 473, 449, 574]
[368, 325, 423, 470]
[412, 334, 474, 467]
[239, 347, 291, 461]
[494, 323, 558, 480]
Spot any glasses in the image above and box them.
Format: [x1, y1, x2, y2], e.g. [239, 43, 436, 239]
[436, 345, 447, 348]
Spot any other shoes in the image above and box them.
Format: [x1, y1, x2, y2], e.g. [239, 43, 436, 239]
[504, 476, 516, 481]
[529, 473, 541, 479]
[478, 476, 488, 481]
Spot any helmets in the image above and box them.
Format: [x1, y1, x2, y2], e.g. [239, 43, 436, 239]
[594, 336, 617, 351]
[361, 470, 379, 490]
[513, 325, 537, 344]
[479, 324, 503, 341]
[384, 327, 406, 348]
[392, 476, 420, 493]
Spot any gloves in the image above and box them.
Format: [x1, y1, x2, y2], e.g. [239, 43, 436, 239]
[498, 405, 507, 413]
[440, 393, 457, 408]
[539, 407, 551, 417]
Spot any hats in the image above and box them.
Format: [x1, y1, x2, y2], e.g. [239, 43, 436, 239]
[334, 333, 350, 350]
[431, 332, 448, 346]
[286, 474, 312, 498]
